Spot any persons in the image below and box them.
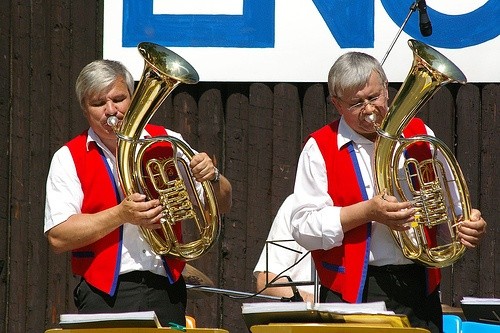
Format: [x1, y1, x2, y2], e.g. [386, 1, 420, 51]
[292, 52, 485, 331]
[254, 194, 320, 306]
[42, 60, 233, 325]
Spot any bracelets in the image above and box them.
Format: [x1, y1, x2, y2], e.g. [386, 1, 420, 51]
[209, 166, 220, 183]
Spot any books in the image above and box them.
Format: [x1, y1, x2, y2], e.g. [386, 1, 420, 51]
[459, 297, 500, 325]
[60, 311, 162, 329]
[241, 299, 413, 332]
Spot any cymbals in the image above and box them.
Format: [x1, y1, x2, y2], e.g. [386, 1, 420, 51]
[180, 263, 215, 296]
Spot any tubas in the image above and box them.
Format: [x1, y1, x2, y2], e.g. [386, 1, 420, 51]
[107, 41, 222, 262]
[364, 38, 472, 270]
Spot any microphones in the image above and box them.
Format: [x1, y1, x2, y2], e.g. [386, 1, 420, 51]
[287, 276, 303, 302]
[416, 0, 432, 37]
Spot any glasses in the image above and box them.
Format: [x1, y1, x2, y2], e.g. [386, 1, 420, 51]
[338, 80, 384, 112]
